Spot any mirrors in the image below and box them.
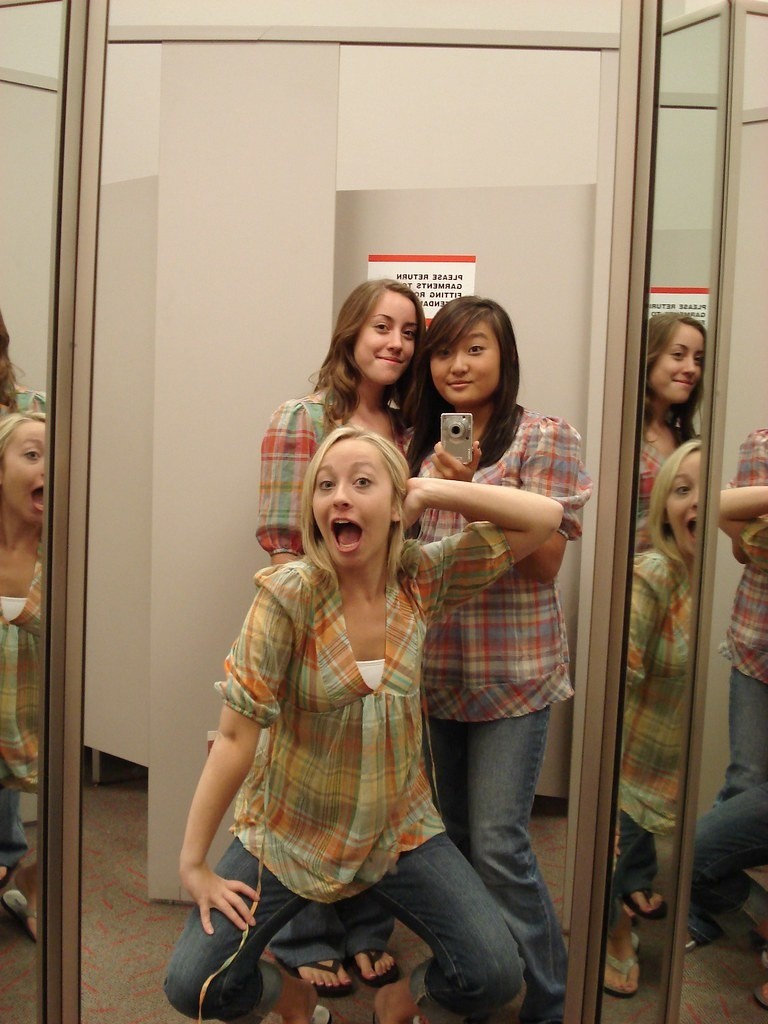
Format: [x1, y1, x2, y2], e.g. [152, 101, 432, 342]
[0, 0, 768, 1024]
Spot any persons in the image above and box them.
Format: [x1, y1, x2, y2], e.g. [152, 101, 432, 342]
[603, 312, 768, 1008]
[0, 309, 46, 943]
[163, 279, 594, 1024]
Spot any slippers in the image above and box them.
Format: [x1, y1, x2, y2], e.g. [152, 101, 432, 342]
[621, 889, 667, 919]
[2, 890, 38, 941]
[350, 950, 400, 988]
[604, 931, 639, 998]
[275, 951, 356, 998]
[752, 982, 768, 1009]
[0, 862, 17, 887]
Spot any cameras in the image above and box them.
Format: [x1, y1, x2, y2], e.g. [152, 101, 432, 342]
[440, 413, 473, 466]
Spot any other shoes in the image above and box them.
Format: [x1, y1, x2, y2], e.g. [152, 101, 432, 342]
[310, 1005, 331, 1024]
